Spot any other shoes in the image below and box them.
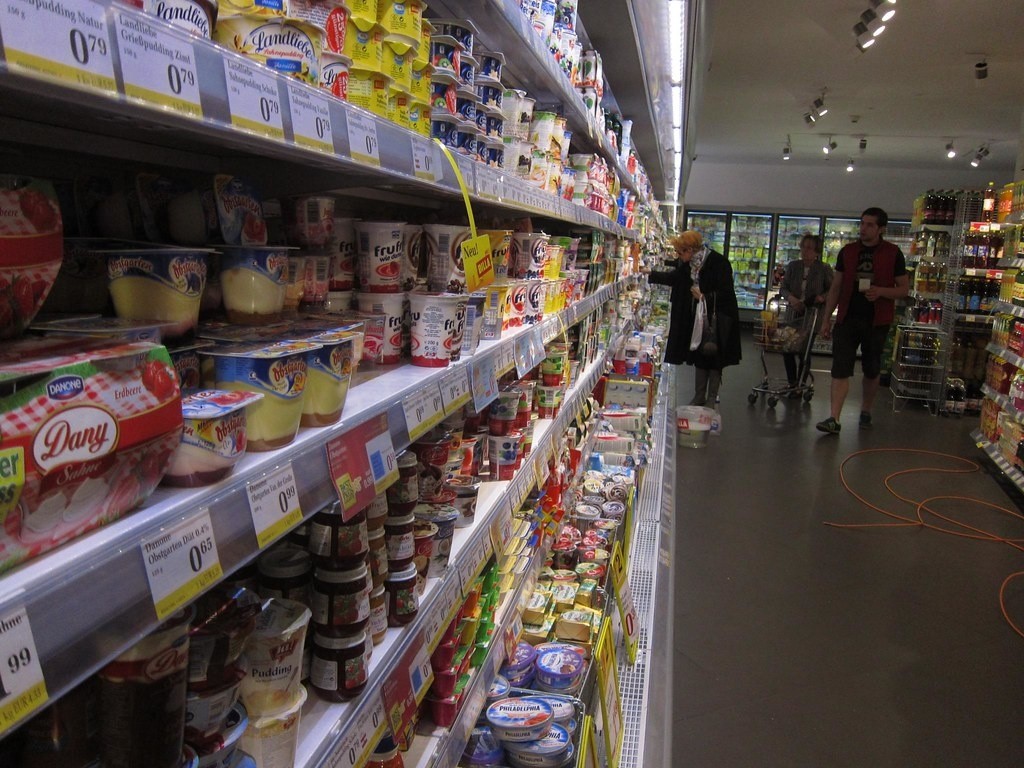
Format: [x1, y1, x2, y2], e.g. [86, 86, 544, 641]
[788, 391, 803, 398]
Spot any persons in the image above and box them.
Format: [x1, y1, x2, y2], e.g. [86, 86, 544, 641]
[816, 206, 910, 435]
[661, 248, 684, 270]
[640, 229, 742, 409]
[779, 235, 835, 399]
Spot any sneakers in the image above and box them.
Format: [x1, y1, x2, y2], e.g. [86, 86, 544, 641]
[816, 417, 841, 434]
[859, 411, 872, 429]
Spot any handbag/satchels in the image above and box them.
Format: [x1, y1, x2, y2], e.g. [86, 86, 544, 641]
[690, 294, 708, 351]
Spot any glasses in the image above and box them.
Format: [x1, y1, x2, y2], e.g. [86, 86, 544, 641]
[674, 249, 687, 255]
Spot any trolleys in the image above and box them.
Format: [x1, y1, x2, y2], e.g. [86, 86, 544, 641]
[746, 293, 826, 407]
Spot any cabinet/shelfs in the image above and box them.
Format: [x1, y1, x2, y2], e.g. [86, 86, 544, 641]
[0, 0, 704, 768]
[970, 179, 1024, 492]
[877, 189, 984, 398]
[928, 188, 1001, 414]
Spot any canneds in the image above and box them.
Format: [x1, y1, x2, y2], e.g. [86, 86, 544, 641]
[245, 450, 420, 702]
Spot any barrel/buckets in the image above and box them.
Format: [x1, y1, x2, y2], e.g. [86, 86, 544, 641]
[676, 406, 714, 448]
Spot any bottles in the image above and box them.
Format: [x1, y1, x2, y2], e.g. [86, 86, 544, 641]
[906, 262, 948, 293]
[911, 180, 1024, 226]
[946, 314, 1024, 475]
[914, 300, 942, 326]
[900, 327, 936, 389]
[909, 227, 951, 258]
[956, 226, 1024, 312]
